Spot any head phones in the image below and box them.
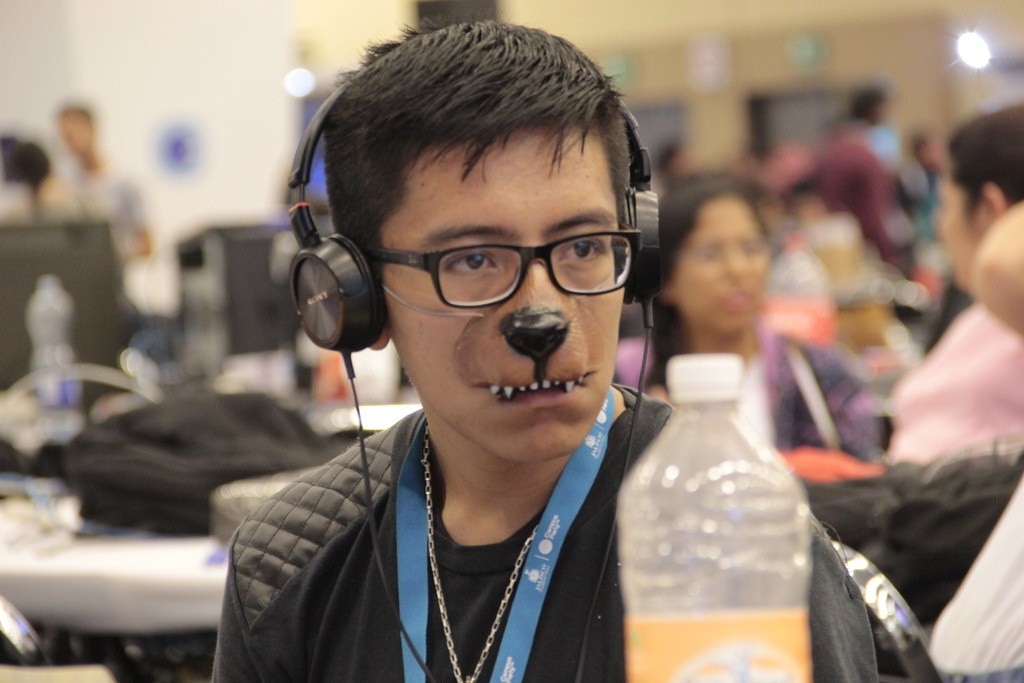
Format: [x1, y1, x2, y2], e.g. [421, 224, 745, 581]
[285, 47, 663, 351]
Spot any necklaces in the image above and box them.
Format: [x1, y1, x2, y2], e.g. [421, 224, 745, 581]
[424, 417, 540, 683]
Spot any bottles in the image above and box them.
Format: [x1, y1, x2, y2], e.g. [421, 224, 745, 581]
[615, 351, 816, 682]
[23, 274, 84, 412]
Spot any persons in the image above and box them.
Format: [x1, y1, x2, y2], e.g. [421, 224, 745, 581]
[1, 100, 154, 256]
[606, 167, 880, 485]
[642, 80, 945, 246]
[882, 101, 1024, 466]
[207, 16, 879, 683]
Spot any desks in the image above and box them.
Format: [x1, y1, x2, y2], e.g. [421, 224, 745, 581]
[0, 400, 425, 640]
